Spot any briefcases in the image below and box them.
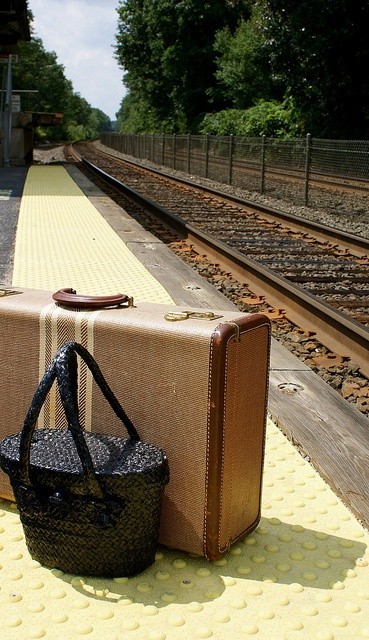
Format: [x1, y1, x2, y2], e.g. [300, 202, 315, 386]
[0, 288, 272, 562]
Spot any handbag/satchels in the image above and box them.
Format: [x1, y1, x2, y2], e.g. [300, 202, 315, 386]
[0, 342, 171, 578]
